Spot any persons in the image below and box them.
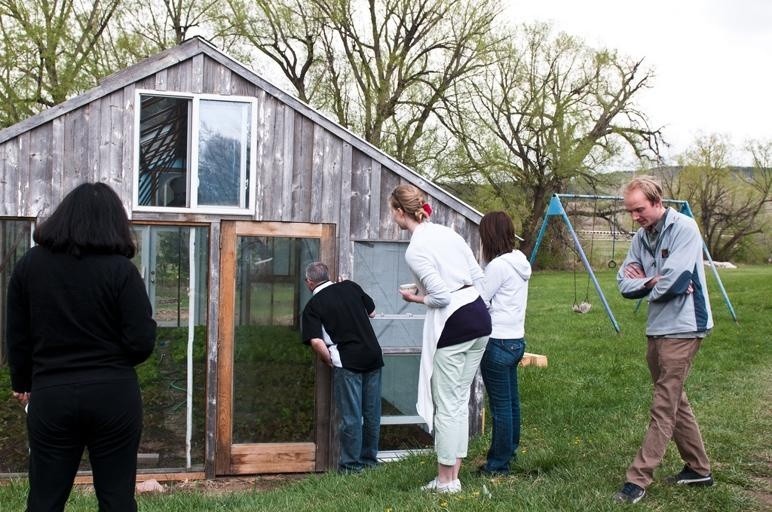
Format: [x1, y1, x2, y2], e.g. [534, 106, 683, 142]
[6, 182, 158, 511]
[609, 174, 715, 505]
[300, 261, 387, 473]
[468, 211, 534, 477]
[387, 186, 493, 494]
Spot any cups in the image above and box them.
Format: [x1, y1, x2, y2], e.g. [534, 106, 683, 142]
[401, 284, 419, 296]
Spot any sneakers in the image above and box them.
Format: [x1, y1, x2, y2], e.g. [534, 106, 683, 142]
[420, 477, 461, 493]
[612, 482, 645, 505]
[664, 464, 713, 487]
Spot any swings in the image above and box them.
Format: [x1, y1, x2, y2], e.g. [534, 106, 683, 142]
[608, 198, 617, 270]
[572, 197, 597, 313]
